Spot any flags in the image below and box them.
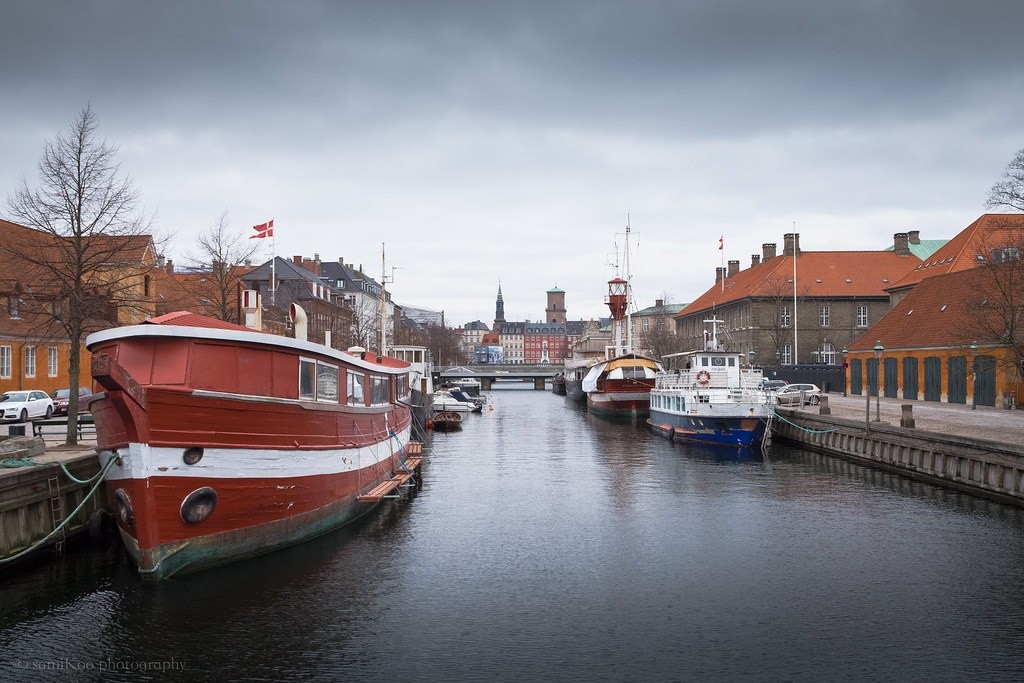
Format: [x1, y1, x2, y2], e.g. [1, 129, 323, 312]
[250, 219, 274, 239]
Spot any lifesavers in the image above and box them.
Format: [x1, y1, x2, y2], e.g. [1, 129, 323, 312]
[696, 370, 711, 384]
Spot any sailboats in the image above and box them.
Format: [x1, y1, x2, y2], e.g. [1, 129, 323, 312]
[582, 213, 667, 419]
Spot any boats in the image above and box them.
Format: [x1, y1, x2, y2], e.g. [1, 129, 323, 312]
[83, 287, 425, 589]
[432, 402, 463, 431]
[552, 374, 566, 395]
[431, 378, 487, 414]
[646, 301, 780, 456]
[564, 358, 598, 403]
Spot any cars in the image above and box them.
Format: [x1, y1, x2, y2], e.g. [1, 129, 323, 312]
[762, 381, 787, 391]
[773, 384, 823, 405]
[0, 389, 55, 423]
[49, 387, 92, 415]
